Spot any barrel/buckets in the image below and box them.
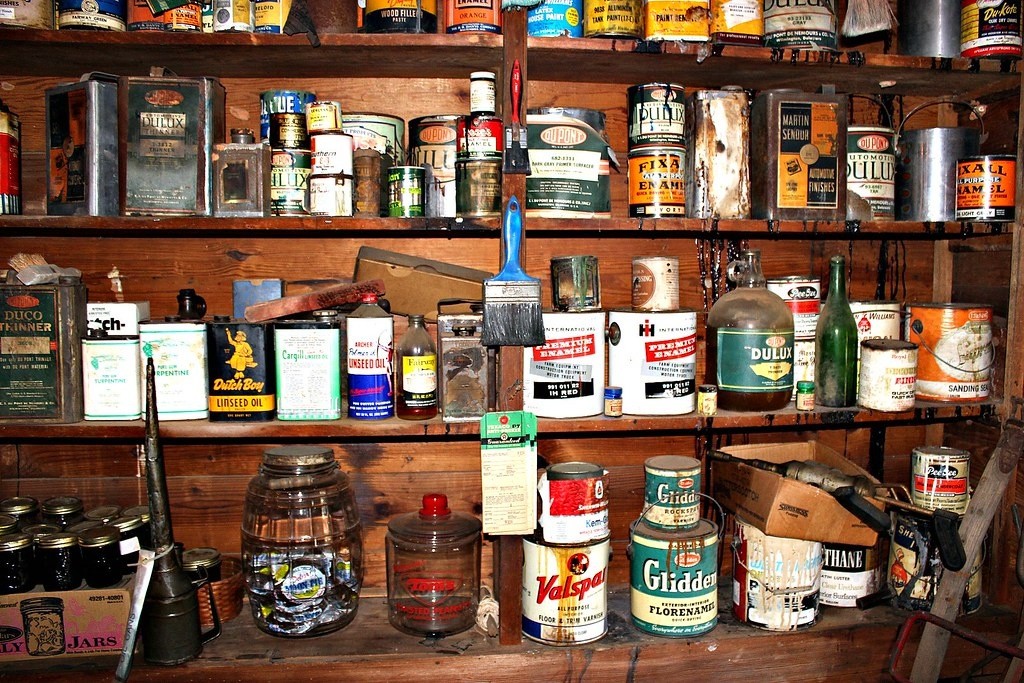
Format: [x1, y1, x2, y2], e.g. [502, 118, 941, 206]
[522, 533, 610, 647]
[903, 302, 994, 402]
[730, 514, 823, 631]
[624, 491, 723, 634]
[895, 98, 983, 220]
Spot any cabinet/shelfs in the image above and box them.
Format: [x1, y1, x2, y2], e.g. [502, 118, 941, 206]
[0, 0, 1024, 683]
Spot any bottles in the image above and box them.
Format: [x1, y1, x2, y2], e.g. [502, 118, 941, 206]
[238, 447, 364, 640]
[705, 249, 793, 411]
[385, 494, 483, 638]
[397, 315, 439, 420]
[814, 255, 857, 407]
[347, 294, 394, 421]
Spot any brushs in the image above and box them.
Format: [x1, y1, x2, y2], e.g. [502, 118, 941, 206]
[480, 193, 546, 347]
